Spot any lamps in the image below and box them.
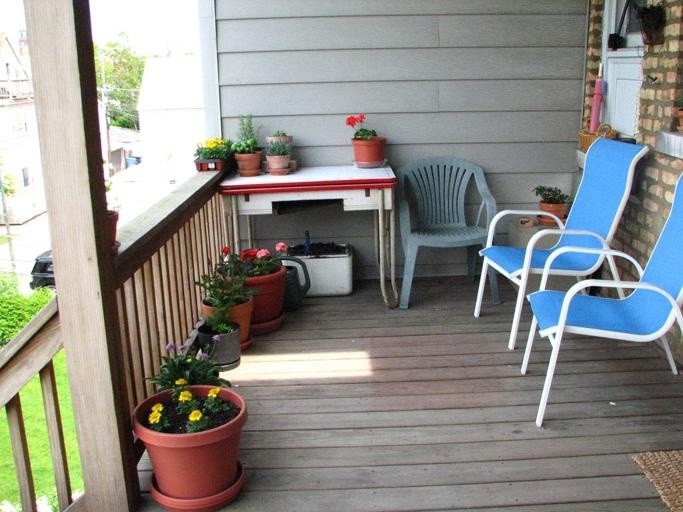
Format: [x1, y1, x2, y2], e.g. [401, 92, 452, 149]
[608, 0, 665, 51]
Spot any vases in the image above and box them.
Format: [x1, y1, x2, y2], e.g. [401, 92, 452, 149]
[193, 156, 229, 171]
[216, 264, 288, 323]
[131, 385, 249, 511]
[351, 136, 386, 166]
[157, 377, 231, 391]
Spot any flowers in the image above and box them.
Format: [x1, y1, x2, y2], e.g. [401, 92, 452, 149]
[148, 333, 225, 392]
[147, 377, 232, 434]
[346, 114, 377, 140]
[215, 242, 289, 277]
[194, 135, 233, 160]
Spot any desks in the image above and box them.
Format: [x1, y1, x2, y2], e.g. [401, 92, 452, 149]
[218, 162, 400, 309]
[507, 218, 587, 299]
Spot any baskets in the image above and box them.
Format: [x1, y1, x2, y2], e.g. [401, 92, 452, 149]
[579, 119, 616, 153]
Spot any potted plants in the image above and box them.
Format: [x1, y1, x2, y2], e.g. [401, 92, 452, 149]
[192, 261, 261, 372]
[532, 183, 573, 227]
[264, 139, 292, 175]
[265, 130, 293, 144]
[230, 111, 263, 176]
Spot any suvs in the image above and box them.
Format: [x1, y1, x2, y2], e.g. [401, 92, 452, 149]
[30, 250, 54, 292]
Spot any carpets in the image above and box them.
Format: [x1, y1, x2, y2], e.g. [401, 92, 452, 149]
[630, 449, 683, 512]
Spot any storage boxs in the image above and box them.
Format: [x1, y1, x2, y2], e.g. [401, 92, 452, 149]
[281, 243, 354, 297]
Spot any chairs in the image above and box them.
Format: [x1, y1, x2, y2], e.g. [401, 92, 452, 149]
[474, 136, 650, 350]
[395, 155, 501, 310]
[520, 171, 682, 428]
